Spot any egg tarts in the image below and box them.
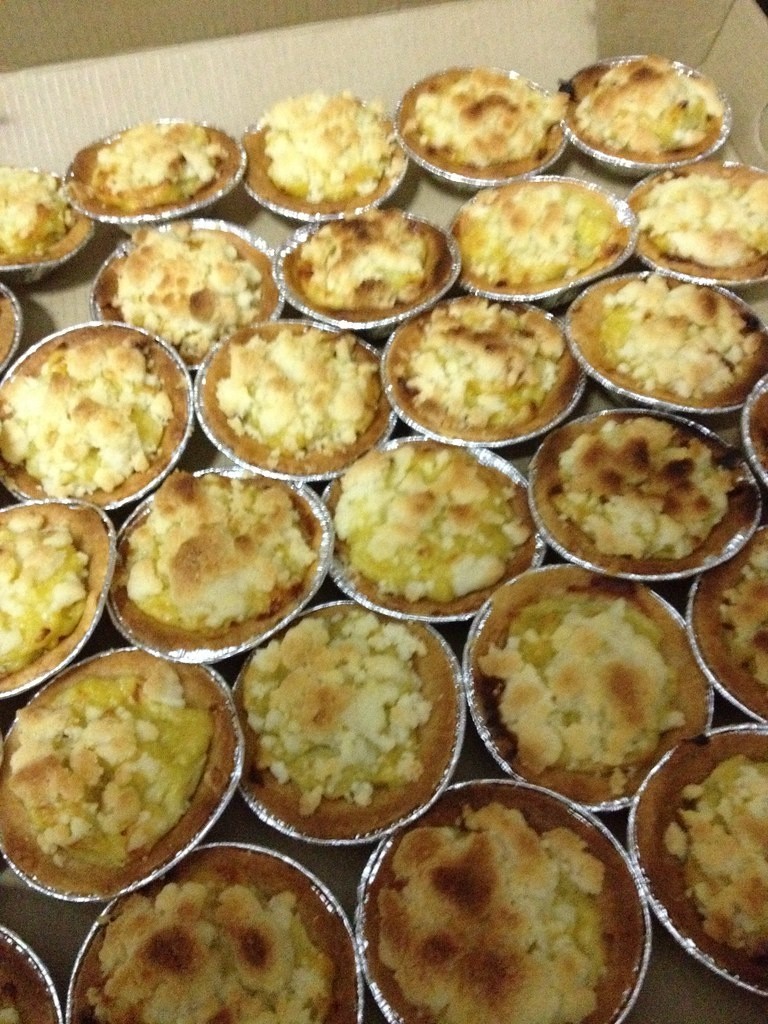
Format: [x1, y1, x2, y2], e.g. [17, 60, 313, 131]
[0, 56, 767, 1024]
